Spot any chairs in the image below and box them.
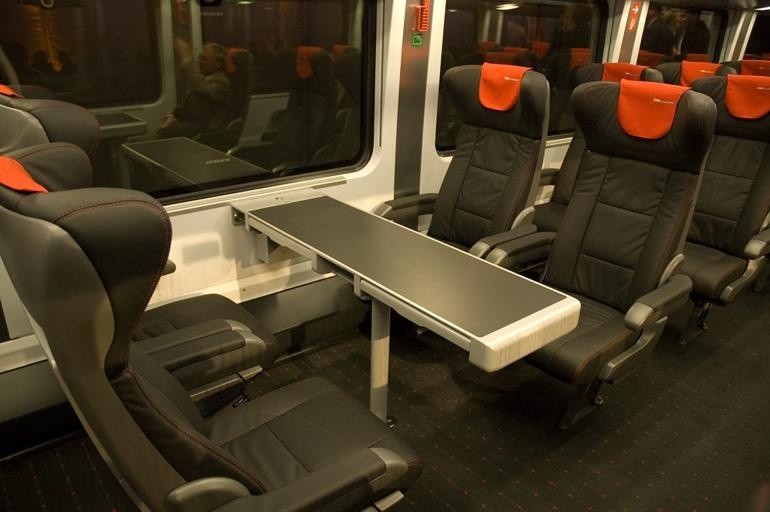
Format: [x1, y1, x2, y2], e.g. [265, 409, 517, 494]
[375, 61, 550, 344]
[1, 144, 421, 511]
[531, 57, 770, 347]
[479, 78, 718, 429]
[1, 90, 281, 416]
[0, 34, 376, 196]
[436, 34, 666, 154]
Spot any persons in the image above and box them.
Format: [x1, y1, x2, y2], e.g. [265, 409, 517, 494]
[126, 43, 237, 145]
[509, 1, 712, 95]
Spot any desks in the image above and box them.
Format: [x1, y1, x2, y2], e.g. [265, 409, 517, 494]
[232, 188, 580, 426]
